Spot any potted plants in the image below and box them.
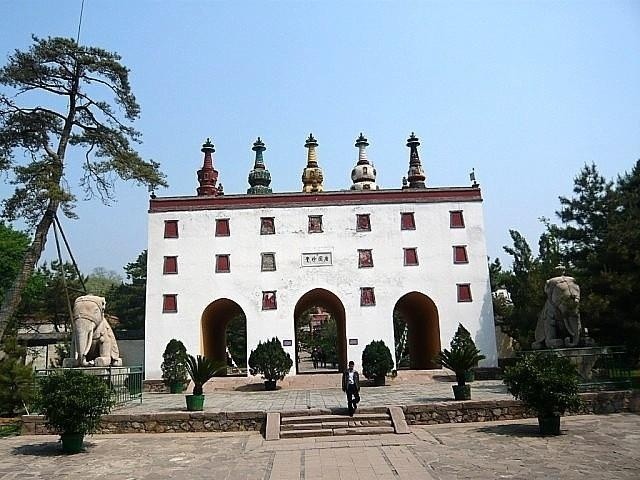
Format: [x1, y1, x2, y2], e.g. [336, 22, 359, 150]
[500, 349, 585, 436]
[35, 369, 117, 451]
[429, 321, 486, 401]
[248, 336, 294, 391]
[361, 339, 394, 386]
[161, 339, 230, 411]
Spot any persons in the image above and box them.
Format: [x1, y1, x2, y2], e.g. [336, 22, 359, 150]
[341, 362, 362, 417]
[296, 342, 338, 370]
